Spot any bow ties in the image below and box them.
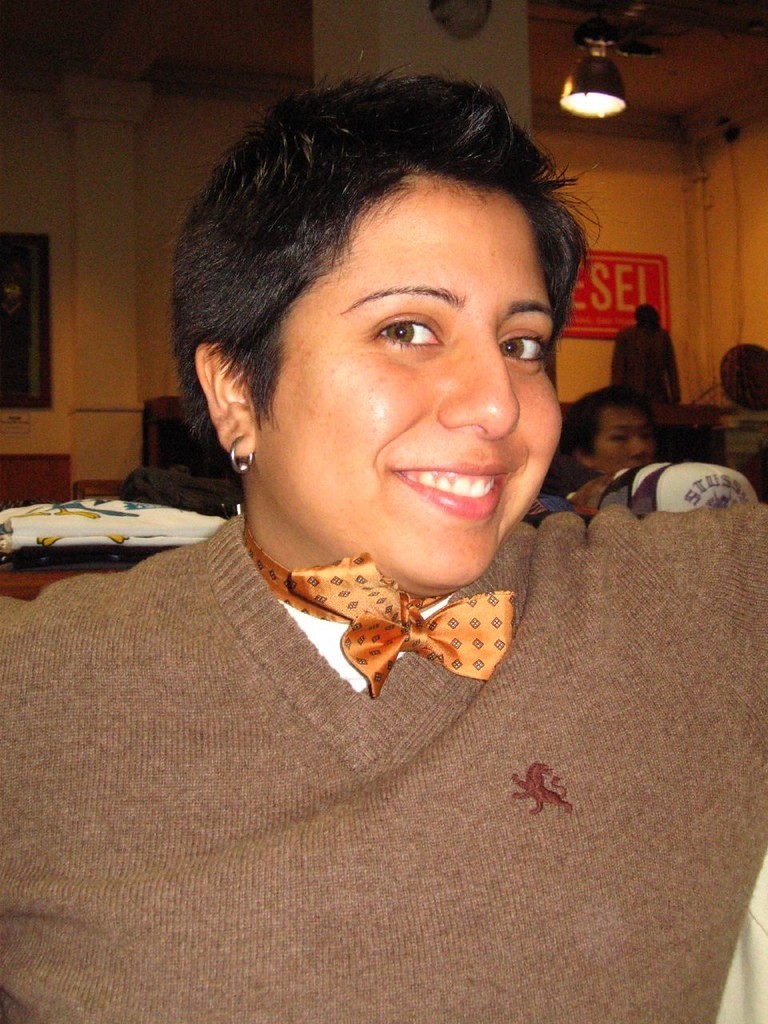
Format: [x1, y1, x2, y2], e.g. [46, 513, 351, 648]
[241, 525, 518, 699]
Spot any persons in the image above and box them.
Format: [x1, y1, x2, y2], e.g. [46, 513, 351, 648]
[612, 305, 682, 403]
[560, 384, 658, 474]
[1, 65, 768, 1023]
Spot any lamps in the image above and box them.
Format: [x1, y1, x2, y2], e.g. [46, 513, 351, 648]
[557, 4, 663, 120]
